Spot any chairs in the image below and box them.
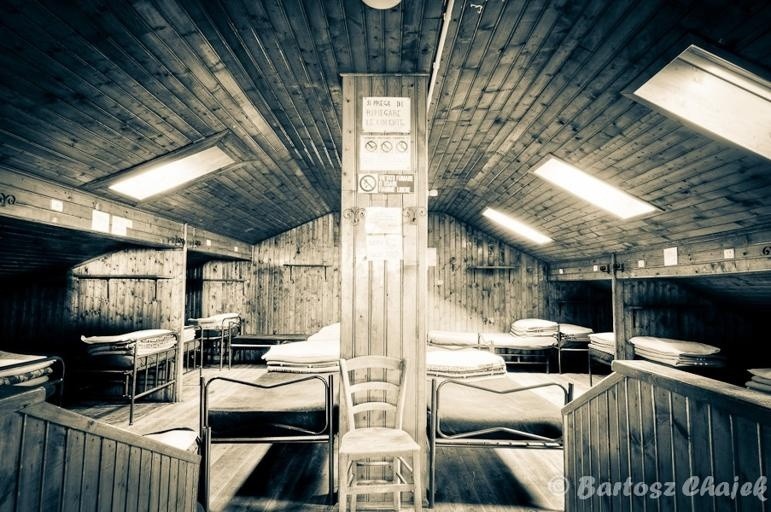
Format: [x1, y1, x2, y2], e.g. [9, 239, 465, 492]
[339, 357, 422, 511]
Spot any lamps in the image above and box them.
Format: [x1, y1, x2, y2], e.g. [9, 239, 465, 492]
[362, 0, 401, 10]
